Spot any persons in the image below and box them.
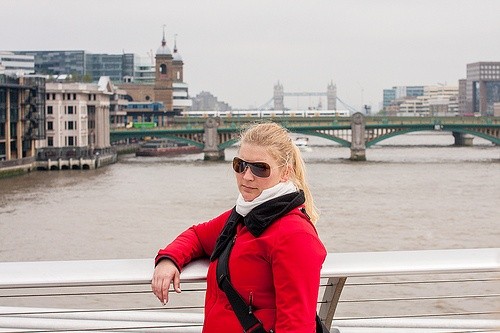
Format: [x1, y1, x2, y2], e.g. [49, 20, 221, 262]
[152, 123, 328, 333]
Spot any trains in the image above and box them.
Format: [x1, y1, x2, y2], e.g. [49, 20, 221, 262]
[179, 110, 350, 117]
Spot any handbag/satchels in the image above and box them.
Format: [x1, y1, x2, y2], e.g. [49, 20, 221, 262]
[316, 311, 330, 333]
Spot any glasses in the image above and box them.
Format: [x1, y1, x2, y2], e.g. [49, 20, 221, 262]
[232, 156, 286, 178]
